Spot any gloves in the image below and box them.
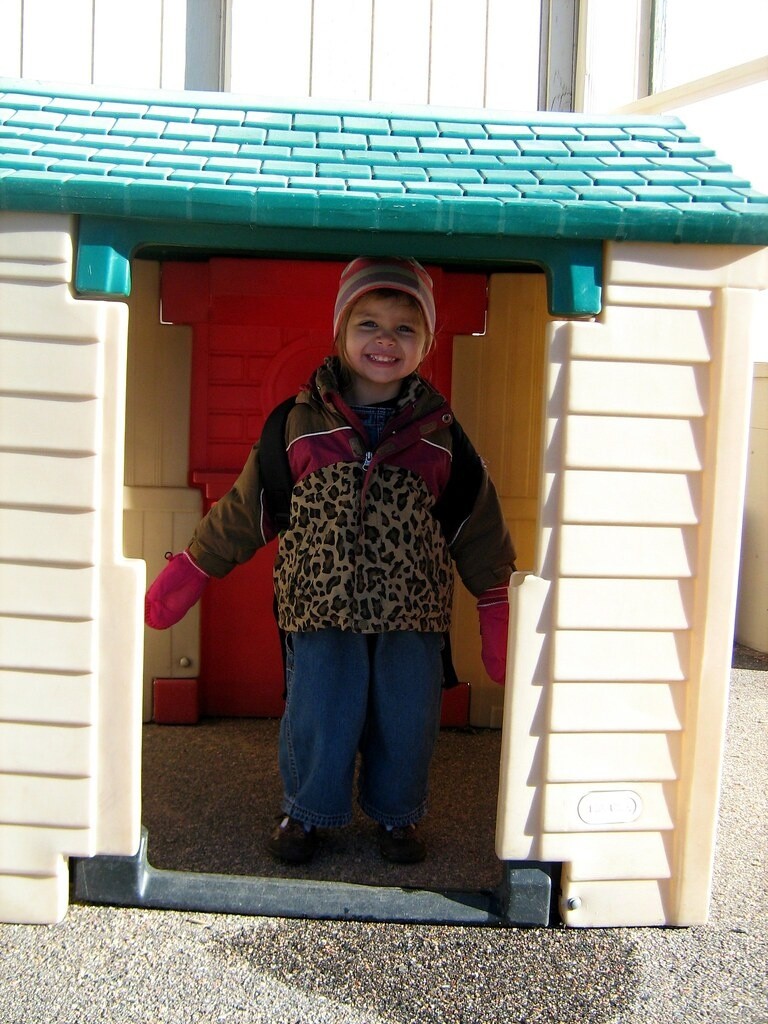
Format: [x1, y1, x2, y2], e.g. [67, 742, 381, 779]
[476, 582, 509, 686]
[144, 548, 212, 631]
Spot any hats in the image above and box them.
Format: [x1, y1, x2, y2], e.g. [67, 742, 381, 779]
[333, 256, 435, 358]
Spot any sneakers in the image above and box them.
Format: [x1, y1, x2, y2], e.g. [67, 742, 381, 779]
[264, 814, 317, 864]
[376, 825, 426, 863]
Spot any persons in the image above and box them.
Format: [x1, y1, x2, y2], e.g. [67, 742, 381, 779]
[144, 256, 519, 867]
